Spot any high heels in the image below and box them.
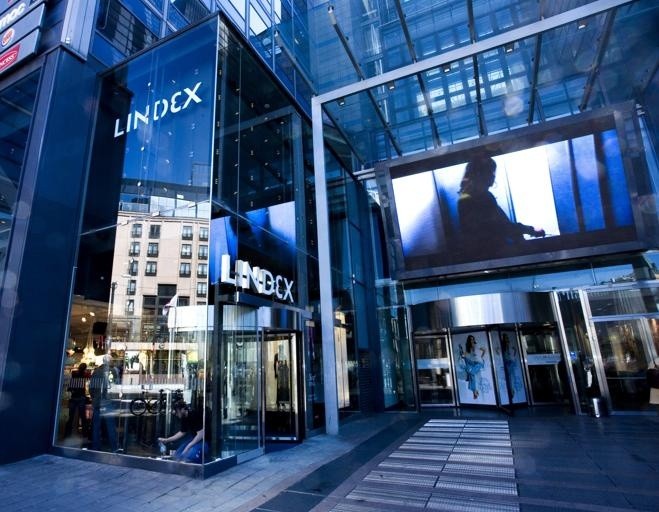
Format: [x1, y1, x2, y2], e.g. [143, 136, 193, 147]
[473, 390, 479, 399]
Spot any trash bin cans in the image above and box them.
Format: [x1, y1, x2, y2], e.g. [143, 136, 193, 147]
[592, 398, 605, 418]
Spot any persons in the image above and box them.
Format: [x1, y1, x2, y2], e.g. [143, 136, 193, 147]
[459, 334, 488, 399]
[495, 332, 523, 402]
[88, 354, 126, 453]
[155, 398, 213, 465]
[64, 362, 93, 434]
[453, 155, 546, 253]
[272, 342, 291, 410]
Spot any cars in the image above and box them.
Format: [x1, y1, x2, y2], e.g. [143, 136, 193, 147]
[397, 377, 452, 403]
[603, 337, 648, 376]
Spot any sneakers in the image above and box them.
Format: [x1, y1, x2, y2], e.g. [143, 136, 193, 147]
[78, 425, 124, 453]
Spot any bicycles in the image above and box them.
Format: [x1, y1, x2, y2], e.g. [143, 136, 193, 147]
[130, 389, 184, 415]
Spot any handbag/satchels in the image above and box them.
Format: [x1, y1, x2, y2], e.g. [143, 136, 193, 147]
[460, 363, 466, 369]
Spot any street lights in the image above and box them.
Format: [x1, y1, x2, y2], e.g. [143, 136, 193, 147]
[102, 274, 131, 398]
[165, 304, 177, 438]
